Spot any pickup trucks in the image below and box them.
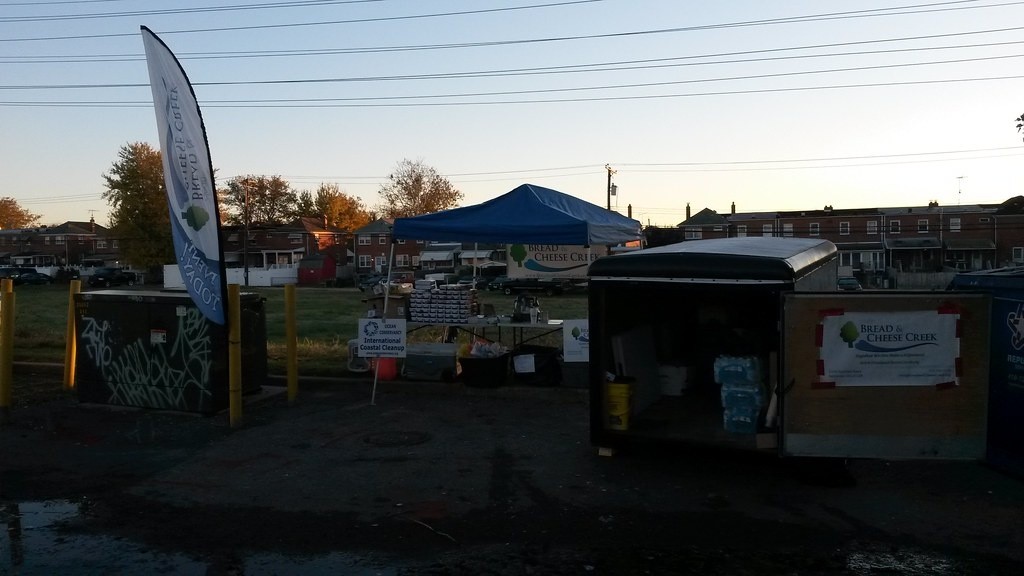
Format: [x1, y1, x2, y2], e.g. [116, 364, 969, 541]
[87, 267, 136, 287]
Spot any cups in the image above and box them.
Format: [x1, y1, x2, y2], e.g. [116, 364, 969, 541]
[530, 308, 538, 324]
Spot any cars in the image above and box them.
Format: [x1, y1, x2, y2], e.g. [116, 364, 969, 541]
[488, 275, 507, 290]
[13, 273, 54, 286]
[836, 276, 862, 291]
[359, 276, 388, 293]
[380, 271, 414, 287]
[940, 260, 971, 271]
[79, 259, 105, 271]
[0, 268, 37, 285]
[457, 275, 487, 290]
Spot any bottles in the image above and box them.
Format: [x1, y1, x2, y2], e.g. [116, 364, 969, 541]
[714, 357, 764, 433]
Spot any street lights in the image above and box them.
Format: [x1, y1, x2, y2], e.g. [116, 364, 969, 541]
[241, 179, 256, 286]
[608, 170, 617, 210]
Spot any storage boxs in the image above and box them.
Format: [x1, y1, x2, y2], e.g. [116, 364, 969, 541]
[348, 337, 512, 388]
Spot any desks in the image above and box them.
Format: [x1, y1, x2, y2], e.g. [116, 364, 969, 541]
[406, 318, 564, 380]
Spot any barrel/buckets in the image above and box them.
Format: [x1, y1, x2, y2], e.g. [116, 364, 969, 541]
[374, 358, 397, 380]
[606, 376, 639, 430]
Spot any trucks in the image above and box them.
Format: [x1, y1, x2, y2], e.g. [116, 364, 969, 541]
[588, 237, 992, 462]
[503, 245, 607, 297]
